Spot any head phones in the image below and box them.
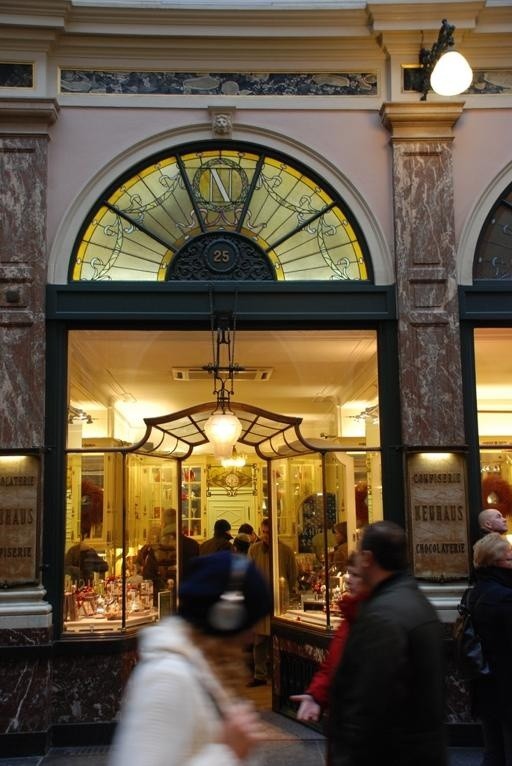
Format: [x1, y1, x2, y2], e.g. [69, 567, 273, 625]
[207, 553, 251, 633]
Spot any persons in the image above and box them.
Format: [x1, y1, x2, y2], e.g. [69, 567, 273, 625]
[472, 531, 511, 766]
[65, 506, 348, 690]
[326, 519, 449, 764]
[477, 507, 509, 535]
[292, 550, 368, 724]
[102, 550, 304, 766]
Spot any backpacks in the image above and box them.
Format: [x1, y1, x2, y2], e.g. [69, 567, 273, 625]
[452, 591, 491, 677]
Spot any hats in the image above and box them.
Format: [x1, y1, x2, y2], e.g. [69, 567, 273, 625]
[179, 553, 271, 635]
[215, 520, 231, 532]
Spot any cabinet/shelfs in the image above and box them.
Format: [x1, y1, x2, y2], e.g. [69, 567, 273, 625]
[259, 458, 346, 549]
[178, 464, 204, 540]
[66, 450, 119, 551]
[123, 453, 178, 555]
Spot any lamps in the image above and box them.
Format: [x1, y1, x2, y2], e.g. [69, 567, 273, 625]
[419, 22, 473, 101]
[204, 285, 248, 468]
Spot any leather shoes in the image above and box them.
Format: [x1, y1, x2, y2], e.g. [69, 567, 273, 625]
[247, 678, 266, 687]
